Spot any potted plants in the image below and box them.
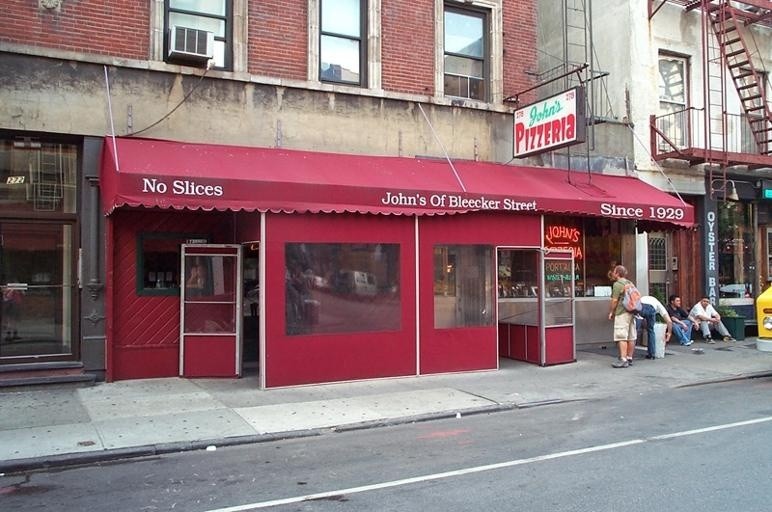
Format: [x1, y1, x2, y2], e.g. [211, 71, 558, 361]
[721, 296, 747, 342]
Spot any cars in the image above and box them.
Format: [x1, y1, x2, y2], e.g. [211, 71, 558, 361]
[300, 269, 378, 302]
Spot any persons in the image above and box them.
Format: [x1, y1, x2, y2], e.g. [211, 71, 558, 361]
[689, 296, 737, 344]
[666, 295, 699, 346]
[247, 283, 259, 319]
[636, 296, 672, 360]
[4, 278, 26, 341]
[607, 264, 642, 368]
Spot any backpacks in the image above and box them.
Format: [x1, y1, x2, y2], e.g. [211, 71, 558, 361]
[616, 280, 642, 314]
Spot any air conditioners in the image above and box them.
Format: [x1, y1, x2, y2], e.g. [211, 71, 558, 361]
[168, 24, 215, 66]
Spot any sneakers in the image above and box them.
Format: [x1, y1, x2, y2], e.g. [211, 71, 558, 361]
[612, 355, 628, 368]
[724, 335, 736, 342]
[681, 339, 694, 346]
[627, 357, 633, 366]
[707, 335, 715, 344]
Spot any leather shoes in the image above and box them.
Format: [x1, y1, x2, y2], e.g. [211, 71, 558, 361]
[645, 354, 655, 360]
